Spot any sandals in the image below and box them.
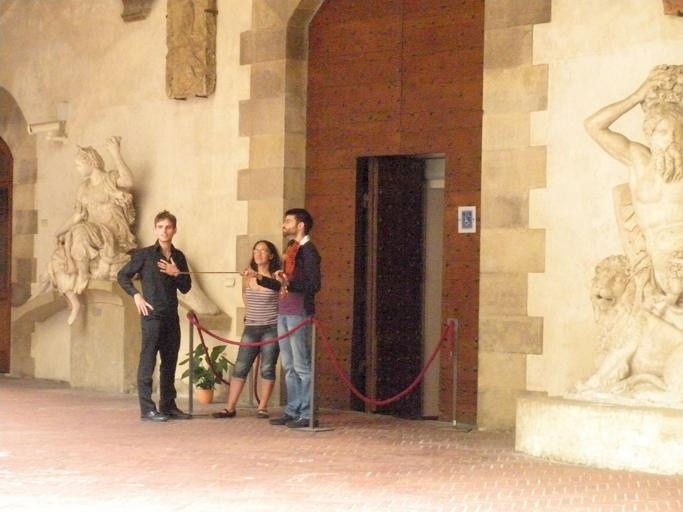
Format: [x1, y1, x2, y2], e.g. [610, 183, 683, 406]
[212, 409, 236, 418]
[256, 409, 269, 418]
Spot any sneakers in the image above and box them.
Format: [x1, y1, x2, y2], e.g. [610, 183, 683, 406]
[270, 414, 319, 428]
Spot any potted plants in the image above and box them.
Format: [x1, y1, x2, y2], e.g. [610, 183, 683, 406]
[178, 344, 227, 406]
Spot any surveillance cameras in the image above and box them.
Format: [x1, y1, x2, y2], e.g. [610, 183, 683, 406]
[27, 120, 67, 143]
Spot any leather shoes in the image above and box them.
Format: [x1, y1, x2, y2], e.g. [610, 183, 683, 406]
[141, 407, 192, 421]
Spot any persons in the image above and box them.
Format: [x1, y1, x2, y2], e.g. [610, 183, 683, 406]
[48, 136, 137, 326]
[117, 209, 193, 422]
[240, 208, 323, 429]
[210, 240, 280, 416]
[585, 61, 682, 316]
